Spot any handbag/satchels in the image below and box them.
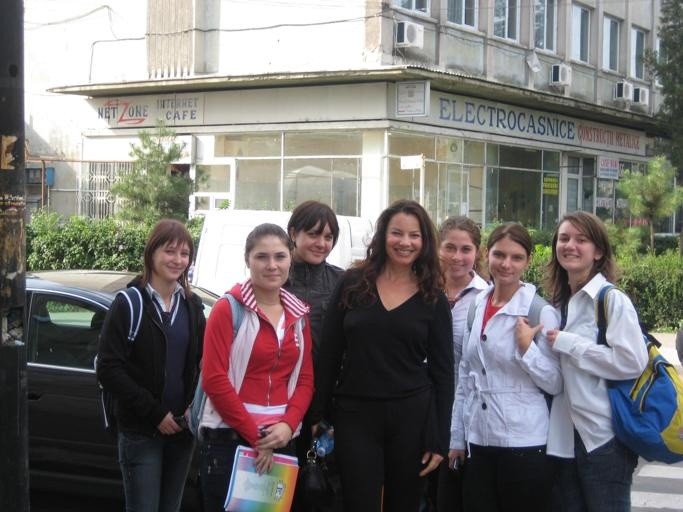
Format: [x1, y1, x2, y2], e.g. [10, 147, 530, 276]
[598, 327, 683, 464]
[298, 458, 343, 511]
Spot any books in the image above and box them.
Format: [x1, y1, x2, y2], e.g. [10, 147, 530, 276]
[223, 446, 301, 512]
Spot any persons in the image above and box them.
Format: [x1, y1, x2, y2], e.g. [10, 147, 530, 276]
[434, 214, 495, 454]
[281, 198, 347, 371]
[194, 222, 317, 511]
[308, 200, 456, 512]
[94, 218, 208, 512]
[544, 209, 649, 512]
[447, 222, 563, 512]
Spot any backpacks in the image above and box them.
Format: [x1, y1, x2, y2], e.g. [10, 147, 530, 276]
[188, 292, 243, 435]
[94, 287, 144, 426]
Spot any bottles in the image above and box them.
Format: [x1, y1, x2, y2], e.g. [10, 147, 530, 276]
[314, 433, 334, 457]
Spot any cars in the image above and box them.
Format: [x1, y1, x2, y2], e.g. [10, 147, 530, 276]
[24, 265, 223, 510]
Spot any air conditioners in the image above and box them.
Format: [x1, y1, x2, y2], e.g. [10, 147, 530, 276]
[611, 78, 635, 103]
[549, 62, 574, 88]
[392, 19, 426, 50]
[632, 84, 651, 106]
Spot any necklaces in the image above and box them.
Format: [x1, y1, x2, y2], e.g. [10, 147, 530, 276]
[255, 297, 280, 305]
